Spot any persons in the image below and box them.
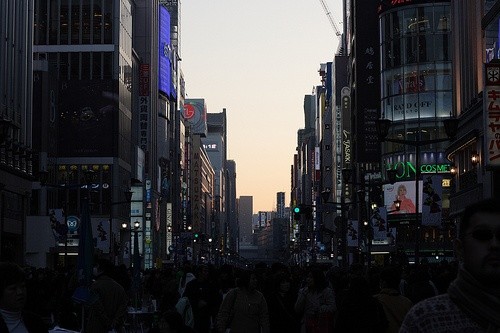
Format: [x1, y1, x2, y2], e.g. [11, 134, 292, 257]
[391, 184, 416, 214]
[400, 198, 500, 333]
[0, 245, 461, 333]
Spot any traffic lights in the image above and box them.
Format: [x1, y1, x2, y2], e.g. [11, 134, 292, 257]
[294, 206, 301, 220]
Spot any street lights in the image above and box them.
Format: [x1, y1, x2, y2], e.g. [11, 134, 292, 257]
[364, 195, 401, 266]
[167, 222, 193, 265]
[194, 236, 212, 262]
[120, 220, 142, 273]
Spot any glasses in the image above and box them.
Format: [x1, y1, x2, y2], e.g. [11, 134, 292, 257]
[466, 228, 500, 243]
